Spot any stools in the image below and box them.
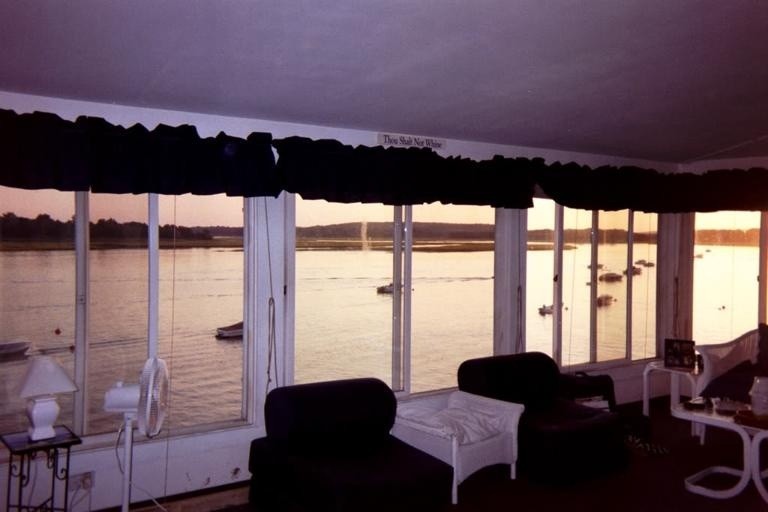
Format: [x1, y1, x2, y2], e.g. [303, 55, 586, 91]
[389, 390, 525, 505]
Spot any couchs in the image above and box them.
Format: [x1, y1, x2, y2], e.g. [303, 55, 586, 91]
[247, 378, 454, 512]
[456, 351, 617, 512]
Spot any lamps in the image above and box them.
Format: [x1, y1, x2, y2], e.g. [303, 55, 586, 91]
[17, 352, 76, 440]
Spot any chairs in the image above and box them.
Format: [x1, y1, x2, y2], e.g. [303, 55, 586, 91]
[690, 325, 768, 447]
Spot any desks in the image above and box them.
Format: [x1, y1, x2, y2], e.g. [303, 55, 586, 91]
[641, 357, 700, 419]
[2, 422, 83, 510]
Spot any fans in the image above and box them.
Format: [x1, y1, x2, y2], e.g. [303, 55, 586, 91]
[104, 357, 173, 512]
